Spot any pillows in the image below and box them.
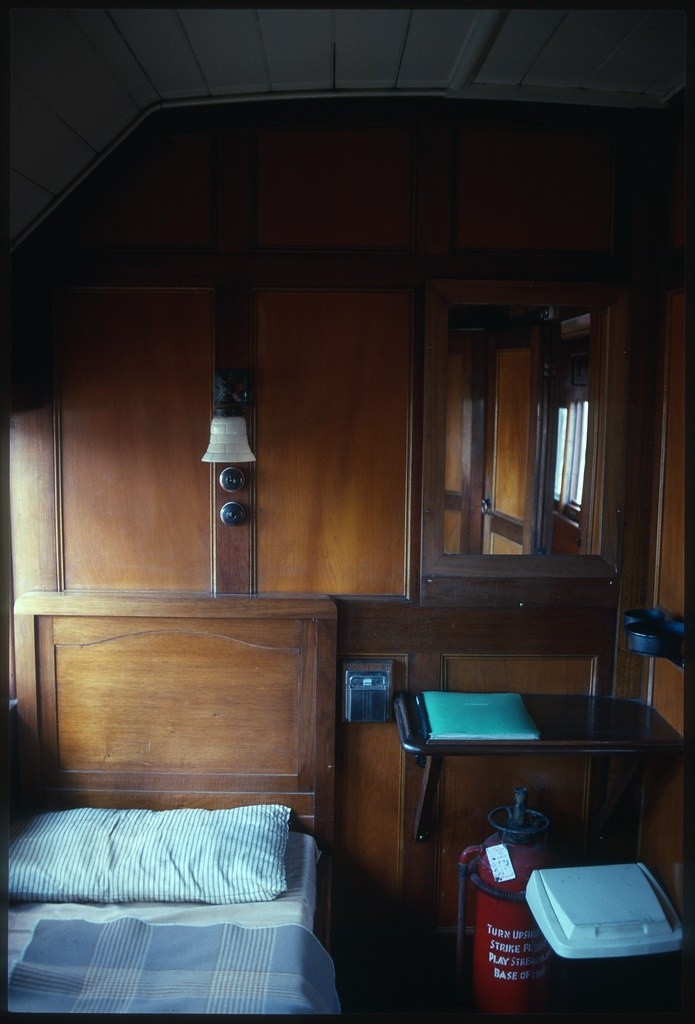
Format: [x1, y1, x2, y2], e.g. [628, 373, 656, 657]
[9, 803, 292, 903]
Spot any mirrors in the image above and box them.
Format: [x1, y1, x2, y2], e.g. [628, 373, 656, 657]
[421, 280, 631, 581]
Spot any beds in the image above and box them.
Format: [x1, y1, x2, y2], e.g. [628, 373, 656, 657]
[10, 598, 345, 1013]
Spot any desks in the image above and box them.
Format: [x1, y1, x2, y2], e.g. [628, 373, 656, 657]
[391, 691, 684, 844]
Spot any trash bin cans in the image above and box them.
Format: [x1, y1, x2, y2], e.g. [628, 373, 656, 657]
[524, 862, 683, 1024]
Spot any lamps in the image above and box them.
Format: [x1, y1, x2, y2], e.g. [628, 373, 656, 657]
[202, 370, 260, 464]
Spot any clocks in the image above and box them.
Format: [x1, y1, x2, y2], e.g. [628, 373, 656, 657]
[570, 352, 591, 385]
